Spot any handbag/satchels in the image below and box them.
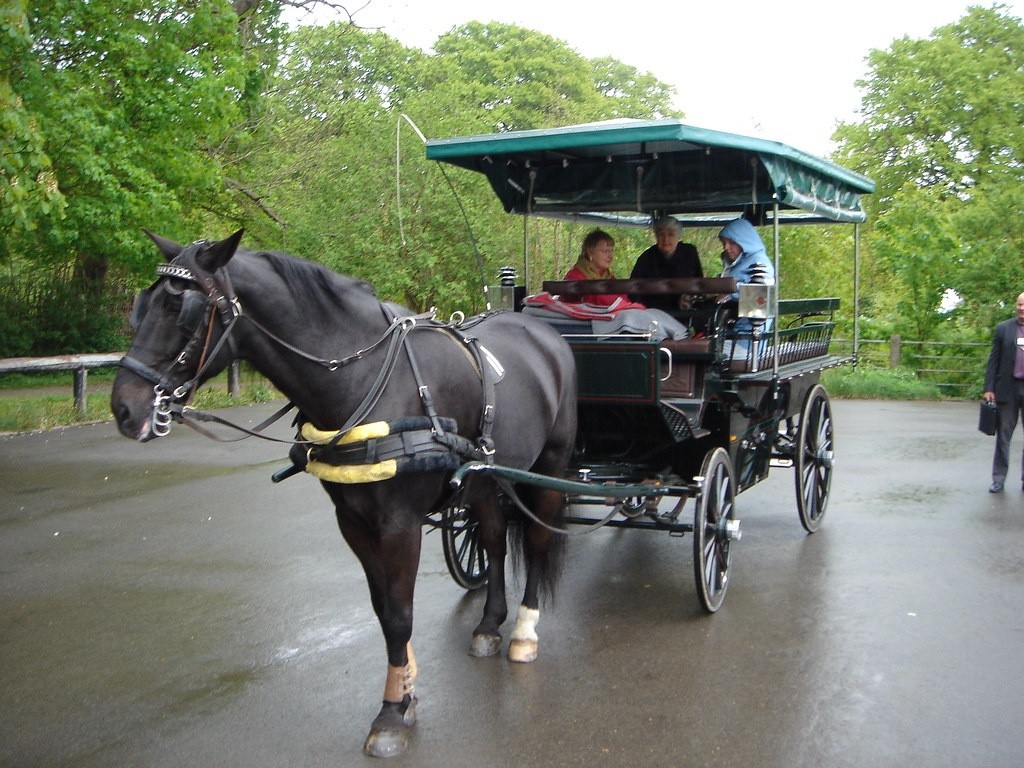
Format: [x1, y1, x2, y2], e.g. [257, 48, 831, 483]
[978, 400, 998, 436]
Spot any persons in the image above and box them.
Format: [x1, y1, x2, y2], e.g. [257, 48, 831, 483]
[558, 230, 628, 307]
[983, 293, 1024, 493]
[709, 219, 775, 361]
[627, 216, 706, 329]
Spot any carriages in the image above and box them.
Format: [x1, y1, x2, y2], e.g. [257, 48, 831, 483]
[111, 112, 875, 760]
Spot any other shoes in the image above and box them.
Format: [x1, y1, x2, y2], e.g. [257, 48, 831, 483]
[990, 482, 1004, 492]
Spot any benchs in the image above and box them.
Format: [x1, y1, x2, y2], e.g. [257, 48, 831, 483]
[774, 297, 840, 322]
[726, 322, 841, 373]
[543, 277, 736, 360]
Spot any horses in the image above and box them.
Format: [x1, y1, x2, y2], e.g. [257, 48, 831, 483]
[111, 224, 580, 757]
